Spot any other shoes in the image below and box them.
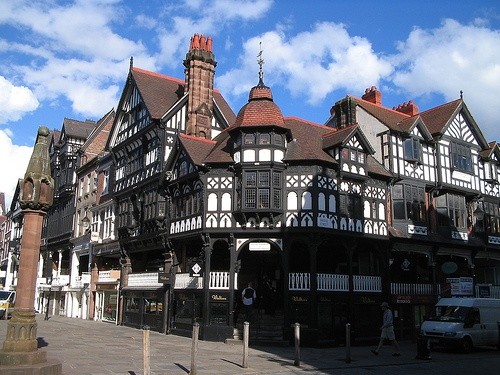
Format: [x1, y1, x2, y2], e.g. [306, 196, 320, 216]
[392, 353, 401, 356]
[371, 350, 378, 356]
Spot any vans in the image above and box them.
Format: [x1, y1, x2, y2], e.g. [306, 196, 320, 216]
[420, 297, 500, 353]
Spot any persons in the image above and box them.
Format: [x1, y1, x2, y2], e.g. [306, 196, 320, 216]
[371, 302, 401, 356]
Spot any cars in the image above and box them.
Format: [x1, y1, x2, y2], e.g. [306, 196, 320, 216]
[0, 290, 17, 319]
